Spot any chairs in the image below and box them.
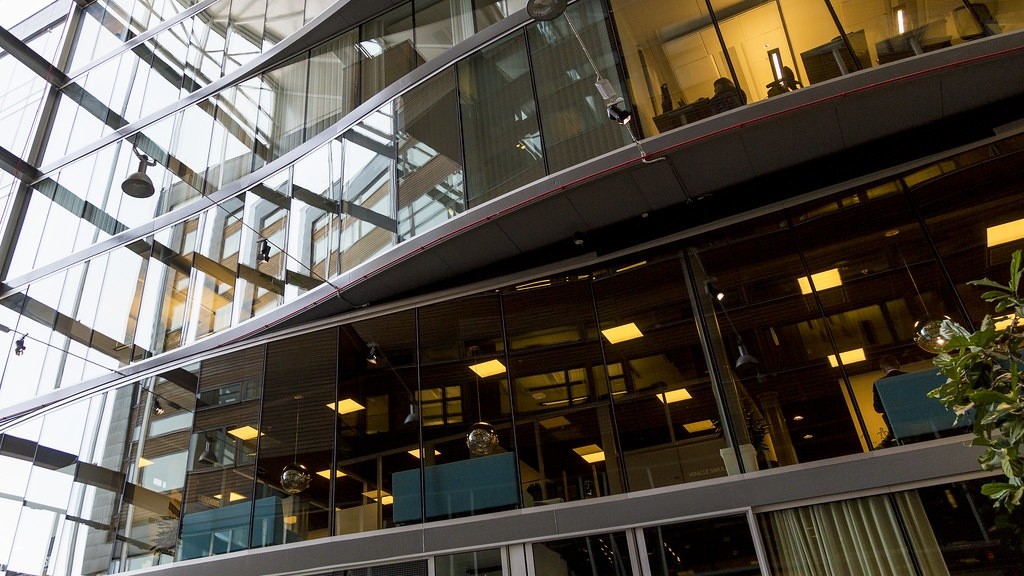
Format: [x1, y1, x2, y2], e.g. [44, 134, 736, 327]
[765, 66, 803, 97]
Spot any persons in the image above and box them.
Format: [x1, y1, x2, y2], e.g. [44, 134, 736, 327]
[872, 354, 911, 437]
[702, 76, 747, 113]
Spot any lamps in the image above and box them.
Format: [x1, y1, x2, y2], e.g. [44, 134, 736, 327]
[280, 392, 314, 493]
[121, 145, 157, 198]
[464, 345, 499, 456]
[256, 238, 271, 264]
[404, 404, 424, 428]
[699, 275, 759, 379]
[366, 337, 381, 365]
[152, 398, 165, 416]
[198, 432, 218, 464]
[880, 228, 956, 354]
[527, 0, 651, 157]
[15, 333, 28, 356]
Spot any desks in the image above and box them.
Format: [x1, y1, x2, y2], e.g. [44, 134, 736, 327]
[652, 97, 714, 134]
[800, 29, 874, 86]
[874, 17, 953, 66]
